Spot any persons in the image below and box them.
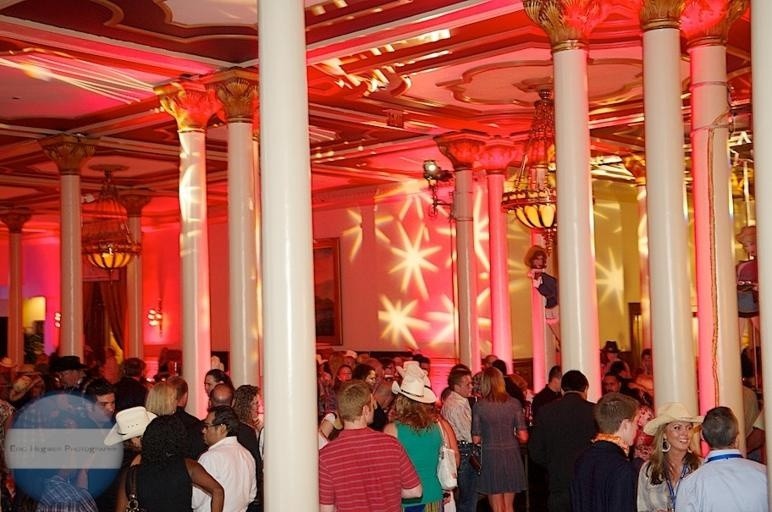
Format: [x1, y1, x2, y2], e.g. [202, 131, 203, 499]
[522, 244, 561, 354]
[734, 225, 760, 341]
[0, 343, 771, 512]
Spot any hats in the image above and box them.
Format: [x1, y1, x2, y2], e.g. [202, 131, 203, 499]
[104, 406, 158, 446]
[344, 350, 358, 359]
[600, 341, 620, 353]
[643, 402, 706, 436]
[0, 356, 88, 401]
[392, 361, 437, 403]
[316, 354, 328, 365]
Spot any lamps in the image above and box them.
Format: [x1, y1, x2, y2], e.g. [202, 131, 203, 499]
[422, 161, 439, 220]
[82, 164, 143, 281]
[500, 78, 558, 256]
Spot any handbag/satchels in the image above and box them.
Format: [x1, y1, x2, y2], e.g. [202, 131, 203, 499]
[125, 490, 144, 512]
[437, 446, 458, 490]
[468, 454, 481, 472]
